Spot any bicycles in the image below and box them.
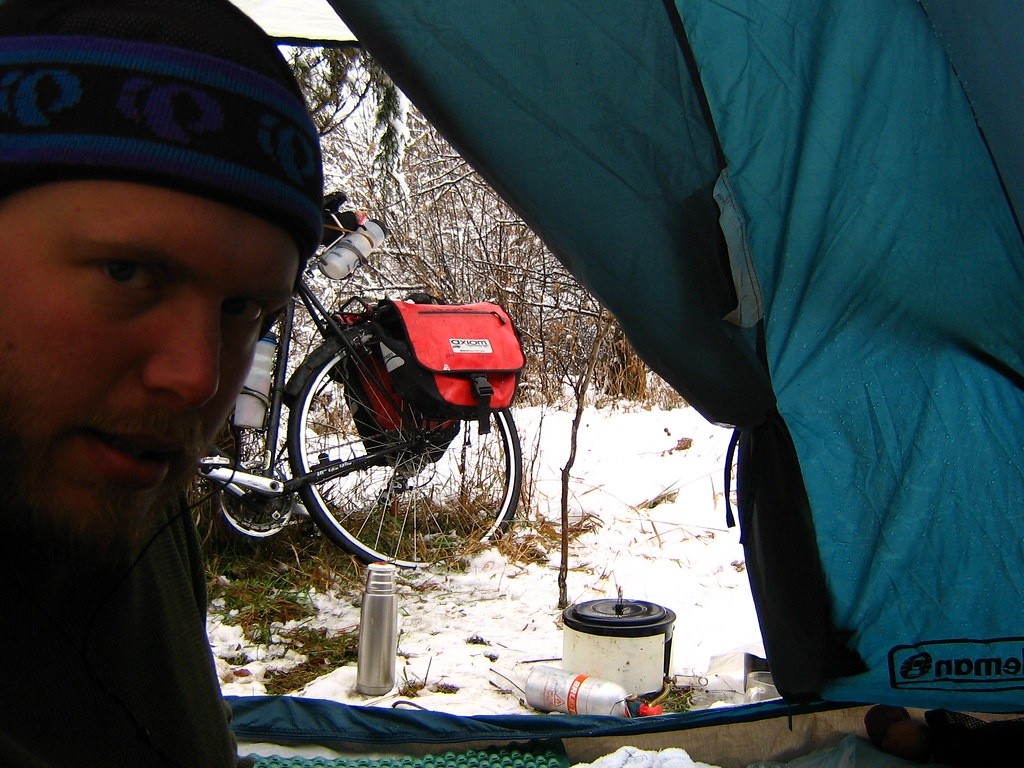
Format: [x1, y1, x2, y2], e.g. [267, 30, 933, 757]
[195, 193, 522, 570]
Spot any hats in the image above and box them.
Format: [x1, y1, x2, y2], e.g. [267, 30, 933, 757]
[0, 0, 323, 283]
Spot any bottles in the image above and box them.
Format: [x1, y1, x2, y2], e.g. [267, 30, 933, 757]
[320, 220, 387, 280]
[523, 666, 664, 718]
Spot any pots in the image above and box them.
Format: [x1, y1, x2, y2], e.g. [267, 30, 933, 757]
[562, 585, 677, 701]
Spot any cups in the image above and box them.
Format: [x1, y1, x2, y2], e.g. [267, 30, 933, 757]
[742, 671, 784, 705]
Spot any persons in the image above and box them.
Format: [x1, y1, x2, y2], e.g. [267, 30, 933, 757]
[0, 0, 326, 768]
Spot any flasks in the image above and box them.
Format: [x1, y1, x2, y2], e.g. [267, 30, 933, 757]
[234, 330, 279, 430]
[357, 563, 400, 696]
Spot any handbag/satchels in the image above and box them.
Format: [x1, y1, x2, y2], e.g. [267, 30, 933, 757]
[373, 297, 527, 436]
[328, 306, 460, 467]
